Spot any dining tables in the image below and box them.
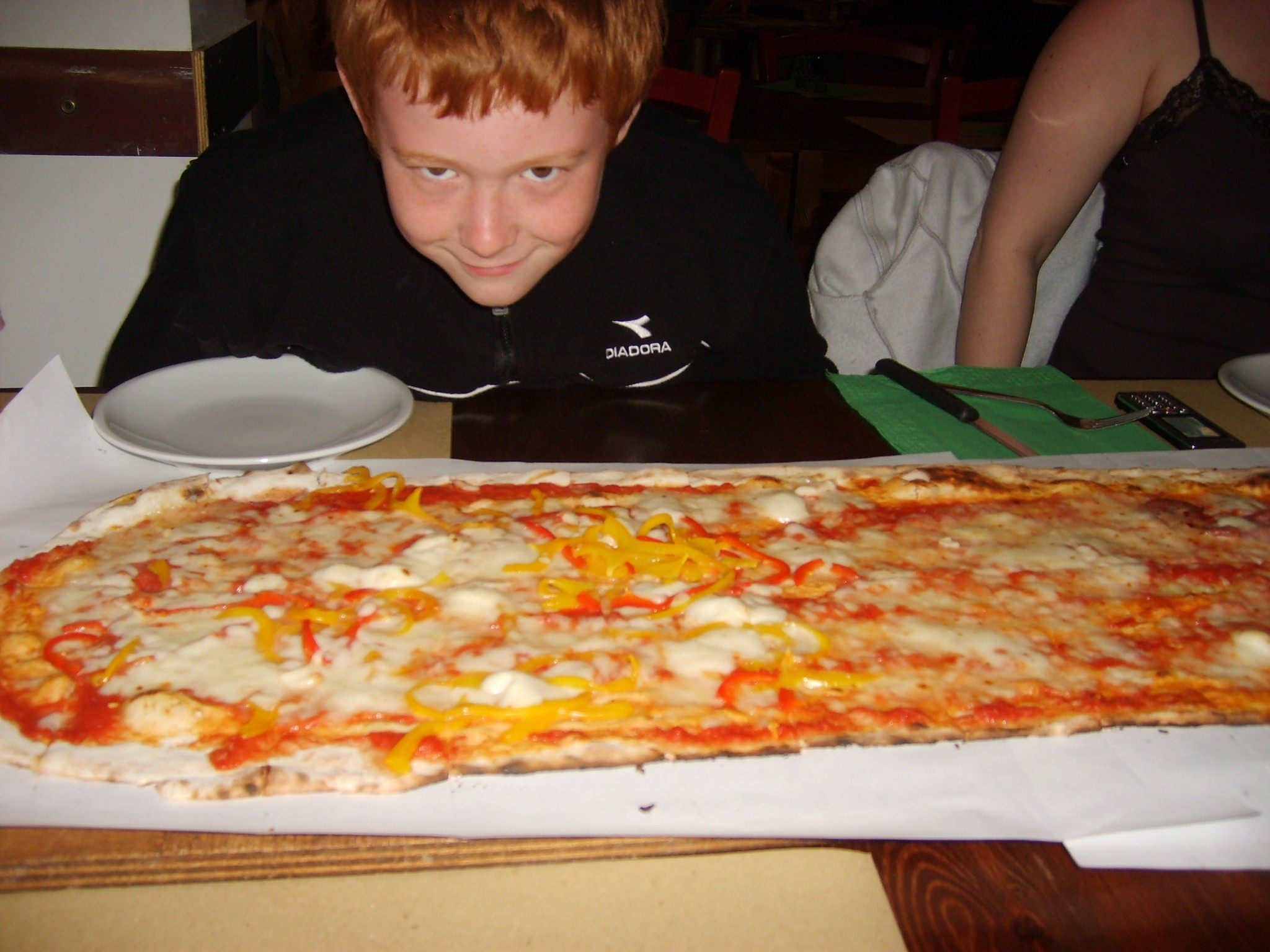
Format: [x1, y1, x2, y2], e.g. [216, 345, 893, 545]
[0, 379, 1268, 952]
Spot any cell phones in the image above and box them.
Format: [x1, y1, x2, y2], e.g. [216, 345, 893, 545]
[1115, 390, 1246, 450]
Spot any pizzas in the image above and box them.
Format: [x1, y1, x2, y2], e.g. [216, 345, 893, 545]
[1, 464, 1269, 798]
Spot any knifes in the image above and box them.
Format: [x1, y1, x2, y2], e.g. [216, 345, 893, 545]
[877, 358, 1035, 457]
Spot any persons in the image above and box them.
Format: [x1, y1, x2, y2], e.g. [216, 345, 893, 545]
[955, 0, 1270, 381]
[98, 0, 839, 400]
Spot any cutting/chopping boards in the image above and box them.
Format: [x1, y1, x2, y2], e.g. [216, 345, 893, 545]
[0, 826, 1270, 952]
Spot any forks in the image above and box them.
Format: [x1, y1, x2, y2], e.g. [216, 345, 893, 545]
[937, 382, 1155, 429]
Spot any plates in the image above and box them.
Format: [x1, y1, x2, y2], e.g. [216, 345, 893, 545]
[94, 353, 414, 471]
[1217, 355, 1270, 415]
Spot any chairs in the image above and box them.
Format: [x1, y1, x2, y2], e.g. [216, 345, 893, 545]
[641, 1, 1028, 287]
